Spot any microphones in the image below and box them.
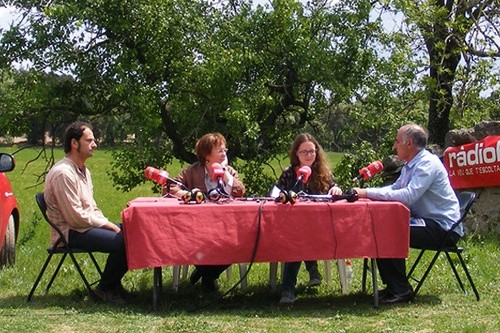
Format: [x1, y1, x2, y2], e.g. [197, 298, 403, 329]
[352, 161, 383, 180]
[210, 161, 225, 188]
[143, 167, 183, 187]
[296, 166, 312, 184]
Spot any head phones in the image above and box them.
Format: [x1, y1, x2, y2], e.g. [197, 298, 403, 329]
[332, 188, 359, 202]
[204, 188, 233, 204]
[177, 191, 206, 204]
[274, 189, 300, 205]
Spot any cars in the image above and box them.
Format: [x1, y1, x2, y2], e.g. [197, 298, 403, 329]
[0, 152, 20, 265]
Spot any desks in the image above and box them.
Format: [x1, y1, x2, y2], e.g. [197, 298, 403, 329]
[120, 197, 410, 307]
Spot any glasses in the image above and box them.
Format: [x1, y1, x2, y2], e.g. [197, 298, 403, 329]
[297, 149, 317, 156]
[210, 148, 228, 154]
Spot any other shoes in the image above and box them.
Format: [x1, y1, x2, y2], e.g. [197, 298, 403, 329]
[308, 267, 322, 286]
[202, 276, 214, 291]
[379, 290, 412, 304]
[379, 285, 413, 297]
[190, 266, 207, 284]
[280, 289, 296, 304]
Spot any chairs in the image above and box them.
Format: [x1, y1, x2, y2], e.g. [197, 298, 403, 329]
[406, 191, 480, 302]
[27, 192, 104, 300]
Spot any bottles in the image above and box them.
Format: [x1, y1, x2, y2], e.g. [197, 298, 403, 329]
[345, 261, 353, 283]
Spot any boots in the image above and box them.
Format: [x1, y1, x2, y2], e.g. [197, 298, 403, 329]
[94, 272, 128, 306]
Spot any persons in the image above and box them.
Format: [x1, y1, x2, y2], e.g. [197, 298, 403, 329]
[44, 121, 140, 306]
[162, 133, 247, 294]
[348, 124, 465, 304]
[269, 133, 344, 301]
